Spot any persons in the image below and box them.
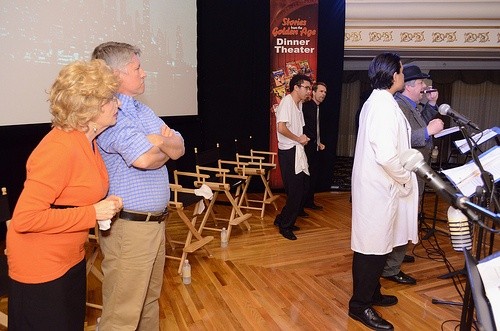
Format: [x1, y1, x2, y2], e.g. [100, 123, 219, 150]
[92, 41, 186, 331]
[380, 65, 445, 285]
[348, 53, 410, 331]
[273, 73, 312, 239]
[6, 59, 123, 331]
[298, 82, 327, 216]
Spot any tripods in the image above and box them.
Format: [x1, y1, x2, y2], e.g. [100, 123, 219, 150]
[418, 127, 462, 240]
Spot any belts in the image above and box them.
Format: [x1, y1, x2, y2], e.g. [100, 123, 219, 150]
[119, 208, 169, 224]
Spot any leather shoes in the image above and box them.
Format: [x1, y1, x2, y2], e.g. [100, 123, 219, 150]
[372, 295, 399, 306]
[384, 271, 416, 284]
[348, 306, 395, 331]
[402, 255, 416, 263]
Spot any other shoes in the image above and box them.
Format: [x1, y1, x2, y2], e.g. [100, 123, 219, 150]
[279, 229, 297, 240]
[310, 204, 323, 210]
[274, 214, 300, 231]
[297, 212, 309, 218]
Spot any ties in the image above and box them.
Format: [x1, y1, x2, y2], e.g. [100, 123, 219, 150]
[316, 105, 320, 152]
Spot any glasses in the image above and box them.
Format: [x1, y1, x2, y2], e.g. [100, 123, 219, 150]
[297, 84, 312, 90]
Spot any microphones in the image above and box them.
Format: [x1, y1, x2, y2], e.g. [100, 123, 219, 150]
[399, 148, 479, 224]
[438, 103, 480, 130]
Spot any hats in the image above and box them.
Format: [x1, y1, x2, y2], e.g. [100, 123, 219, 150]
[403, 64, 431, 82]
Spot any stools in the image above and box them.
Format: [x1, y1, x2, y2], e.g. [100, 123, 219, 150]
[86, 148, 281, 310]
